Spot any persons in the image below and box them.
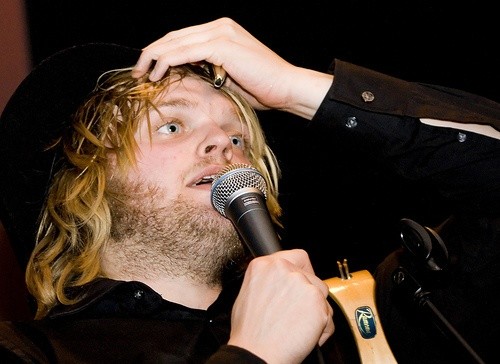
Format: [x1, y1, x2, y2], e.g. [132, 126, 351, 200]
[0, 16, 500, 364]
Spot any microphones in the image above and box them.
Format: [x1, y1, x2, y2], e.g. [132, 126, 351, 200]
[208, 163, 324, 363]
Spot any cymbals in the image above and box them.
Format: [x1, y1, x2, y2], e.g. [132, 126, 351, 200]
[316, 258, 400, 364]
